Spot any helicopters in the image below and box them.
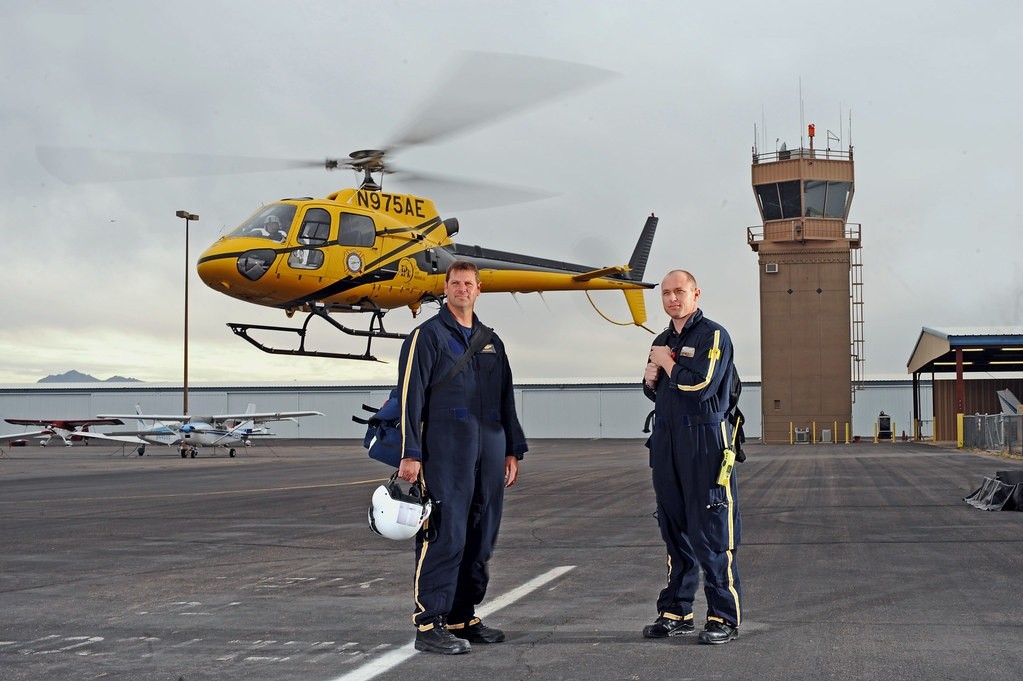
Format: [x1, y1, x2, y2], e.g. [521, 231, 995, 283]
[33, 45, 659, 359]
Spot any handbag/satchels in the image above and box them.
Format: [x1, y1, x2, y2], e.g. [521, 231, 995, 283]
[365, 388, 402, 469]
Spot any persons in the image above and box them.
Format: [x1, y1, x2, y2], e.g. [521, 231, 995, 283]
[641, 269, 744, 646]
[263, 216, 283, 242]
[397, 260, 529, 654]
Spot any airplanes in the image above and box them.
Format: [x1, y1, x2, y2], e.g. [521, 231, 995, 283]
[0, 403, 330, 461]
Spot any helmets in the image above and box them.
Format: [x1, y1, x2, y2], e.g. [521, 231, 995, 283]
[367, 485, 432, 540]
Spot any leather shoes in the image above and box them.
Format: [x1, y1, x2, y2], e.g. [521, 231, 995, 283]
[644, 617, 695, 638]
[414, 627, 471, 654]
[699, 621, 739, 643]
[449, 623, 505, 644]
